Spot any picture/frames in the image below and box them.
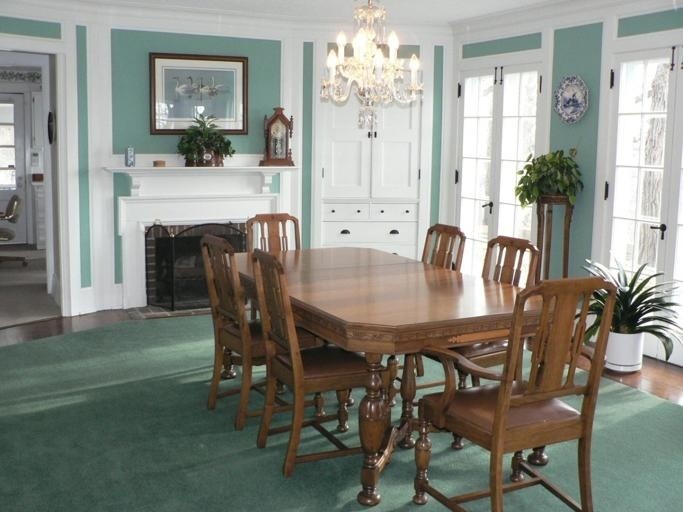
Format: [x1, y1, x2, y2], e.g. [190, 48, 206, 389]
[147, 52, 248, 136]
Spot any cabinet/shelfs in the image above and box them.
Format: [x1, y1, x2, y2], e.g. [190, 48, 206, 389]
[309, 57, 433, 263]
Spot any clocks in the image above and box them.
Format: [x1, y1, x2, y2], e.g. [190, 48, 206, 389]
[259, 106, 295, 167]
[552, 74, 589, 126]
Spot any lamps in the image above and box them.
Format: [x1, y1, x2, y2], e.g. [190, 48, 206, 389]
[318, 2, 424, 108]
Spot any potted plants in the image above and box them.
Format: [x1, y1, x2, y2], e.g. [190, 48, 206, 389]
[573, 256, 682, 373]
[176, 113, 236, 168]
[514, 148, 584, 206]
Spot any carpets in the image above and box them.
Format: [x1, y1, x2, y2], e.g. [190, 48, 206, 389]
[0, 314, 683, 512]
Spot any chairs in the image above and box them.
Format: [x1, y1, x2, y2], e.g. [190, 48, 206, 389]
[0, 194, 28, 267]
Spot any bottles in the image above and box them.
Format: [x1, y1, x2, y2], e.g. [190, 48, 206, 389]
[125, 145, 136, 167]
